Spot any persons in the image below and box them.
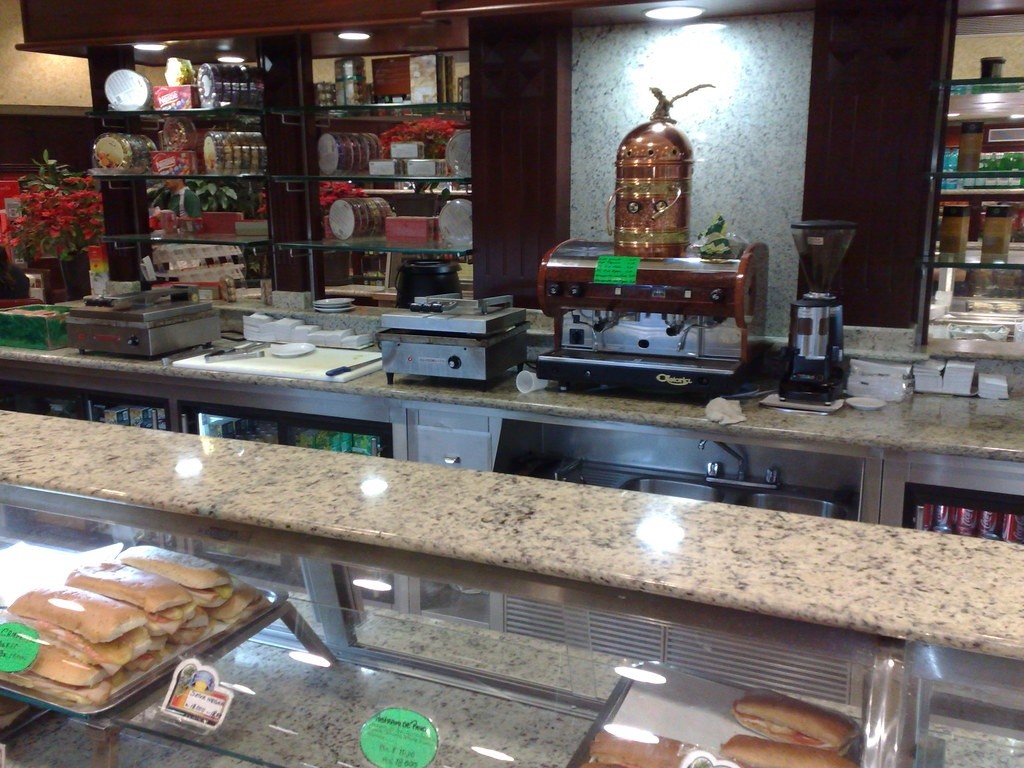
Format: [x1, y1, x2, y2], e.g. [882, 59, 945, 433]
[0, 246, 30, 300]
[160, 177, 201, 231]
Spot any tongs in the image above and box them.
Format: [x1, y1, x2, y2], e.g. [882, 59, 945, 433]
[205, 341, 271, 363]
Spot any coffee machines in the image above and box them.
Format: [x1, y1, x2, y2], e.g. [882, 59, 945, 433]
[777, 218, 858, 407]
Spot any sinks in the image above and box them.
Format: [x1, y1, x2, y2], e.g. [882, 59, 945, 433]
[619, 478, 724, 505]
[737, 492, 849, 520]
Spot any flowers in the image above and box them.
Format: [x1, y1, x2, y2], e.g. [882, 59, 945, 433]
[7, 148, 110, 265]
[381, 111, 465, 198]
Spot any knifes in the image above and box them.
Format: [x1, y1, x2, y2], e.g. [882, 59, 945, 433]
[326, 356, 382, 377]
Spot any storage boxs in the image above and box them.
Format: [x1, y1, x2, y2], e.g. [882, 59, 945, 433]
[0, 304, 73, 350]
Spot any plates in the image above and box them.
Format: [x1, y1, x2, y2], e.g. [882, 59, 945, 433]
[311, 297, 358, 313]
[270, 342, 315, 358]
[845, 396, 888, 411]
[105, 69, 148, 112]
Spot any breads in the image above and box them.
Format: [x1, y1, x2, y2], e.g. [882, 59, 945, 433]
[575, 687, 866, 768]
[0, 544, 268, 698]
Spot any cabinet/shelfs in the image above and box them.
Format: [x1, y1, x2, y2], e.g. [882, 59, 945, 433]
[918, 75, 1024, 341]
[77, 103, 477, 301]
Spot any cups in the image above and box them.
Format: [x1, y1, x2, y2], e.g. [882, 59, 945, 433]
[516, 370, 548, 394]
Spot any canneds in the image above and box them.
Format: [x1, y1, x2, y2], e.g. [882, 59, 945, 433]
[912, 503, 1024, 543]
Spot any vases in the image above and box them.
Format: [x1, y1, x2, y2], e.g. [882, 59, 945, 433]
[69, 238, 93, 295]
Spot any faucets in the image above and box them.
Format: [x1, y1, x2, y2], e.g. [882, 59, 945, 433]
[698, 440, 747, 479]
